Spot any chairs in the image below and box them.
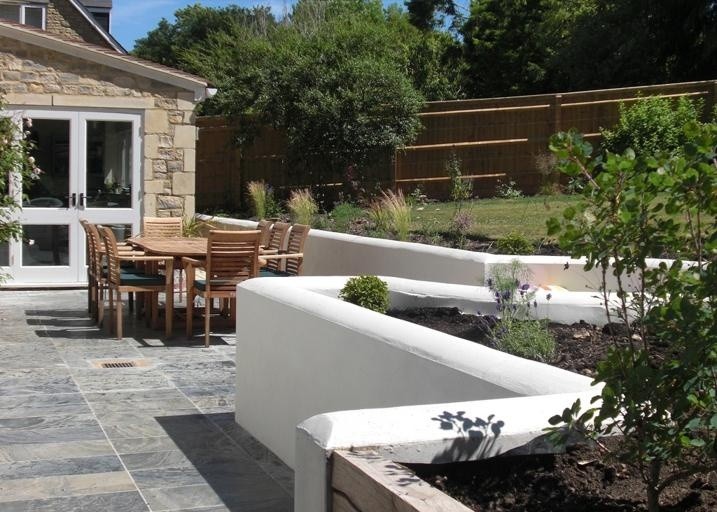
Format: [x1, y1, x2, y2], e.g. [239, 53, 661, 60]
[256, 223, 311, 277]
[141, 216, 183, 319]
[82, 223, 144, 329]
[180, 229, 262, 348]
[256, 218, 271, 241]
[253, 221, 290, 270]
[79, 218, 133, 317]
[97, 224, 174, 339]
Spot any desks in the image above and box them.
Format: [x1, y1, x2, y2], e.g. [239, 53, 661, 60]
[125, 236, 279, 339]
